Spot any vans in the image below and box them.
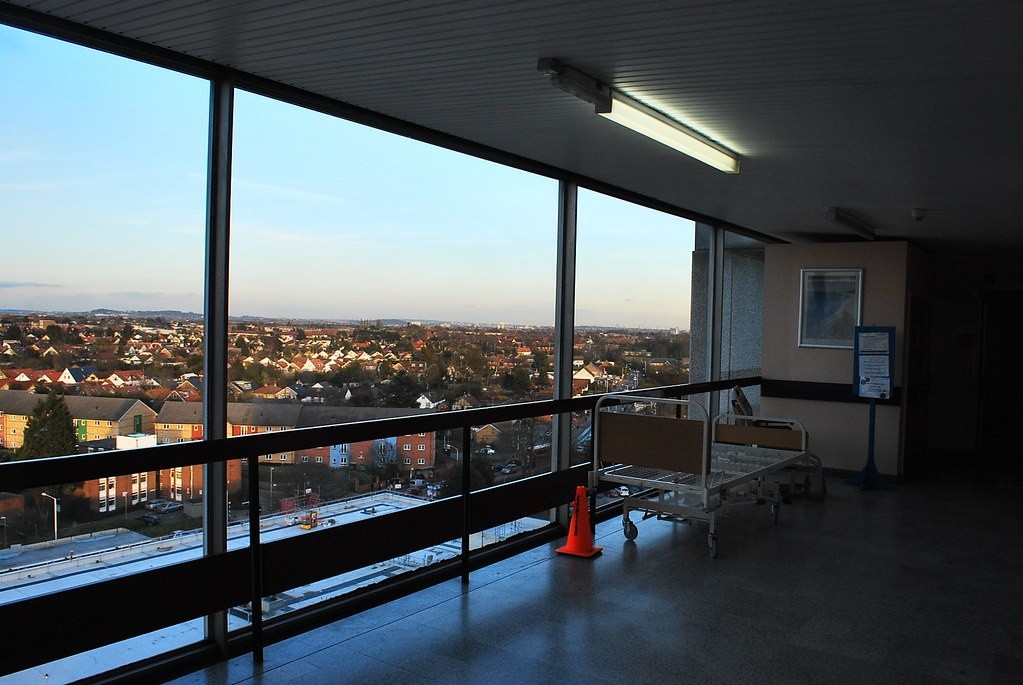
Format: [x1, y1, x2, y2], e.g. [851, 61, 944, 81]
[615, 486, 630, 496]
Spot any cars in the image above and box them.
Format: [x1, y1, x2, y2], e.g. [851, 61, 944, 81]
[142, 514, 160, 527]
[474, 448, 495, 456]
[597, 490, 617, 498]
[145, 498, 184, 514]
[488, 463, 517, 474]
[406, 479, 447, 497]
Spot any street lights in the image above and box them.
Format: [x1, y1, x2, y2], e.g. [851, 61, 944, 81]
[1, 517, 6, 549]
[445, 445, 458, 463]
[270, 467, 274, 499]
[123, 492, 127, 520]
[41, 493, 57, 540]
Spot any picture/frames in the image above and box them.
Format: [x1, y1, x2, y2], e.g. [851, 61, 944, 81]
[798, 267, 863, 348]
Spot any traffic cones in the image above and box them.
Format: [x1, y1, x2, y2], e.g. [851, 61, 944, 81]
[555, 486, 604, 558]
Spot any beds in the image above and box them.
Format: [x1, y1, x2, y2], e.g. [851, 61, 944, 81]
[589, 392, 826, 558]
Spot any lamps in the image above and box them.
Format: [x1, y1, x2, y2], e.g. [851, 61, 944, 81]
[826, 208, 875, 240]
[537, 57, 742, 176]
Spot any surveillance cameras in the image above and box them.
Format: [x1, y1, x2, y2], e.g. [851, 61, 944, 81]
[912, 208, 923, 221]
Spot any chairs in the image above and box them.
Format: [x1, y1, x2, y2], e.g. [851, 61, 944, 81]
[731, 385, 827, 495]
